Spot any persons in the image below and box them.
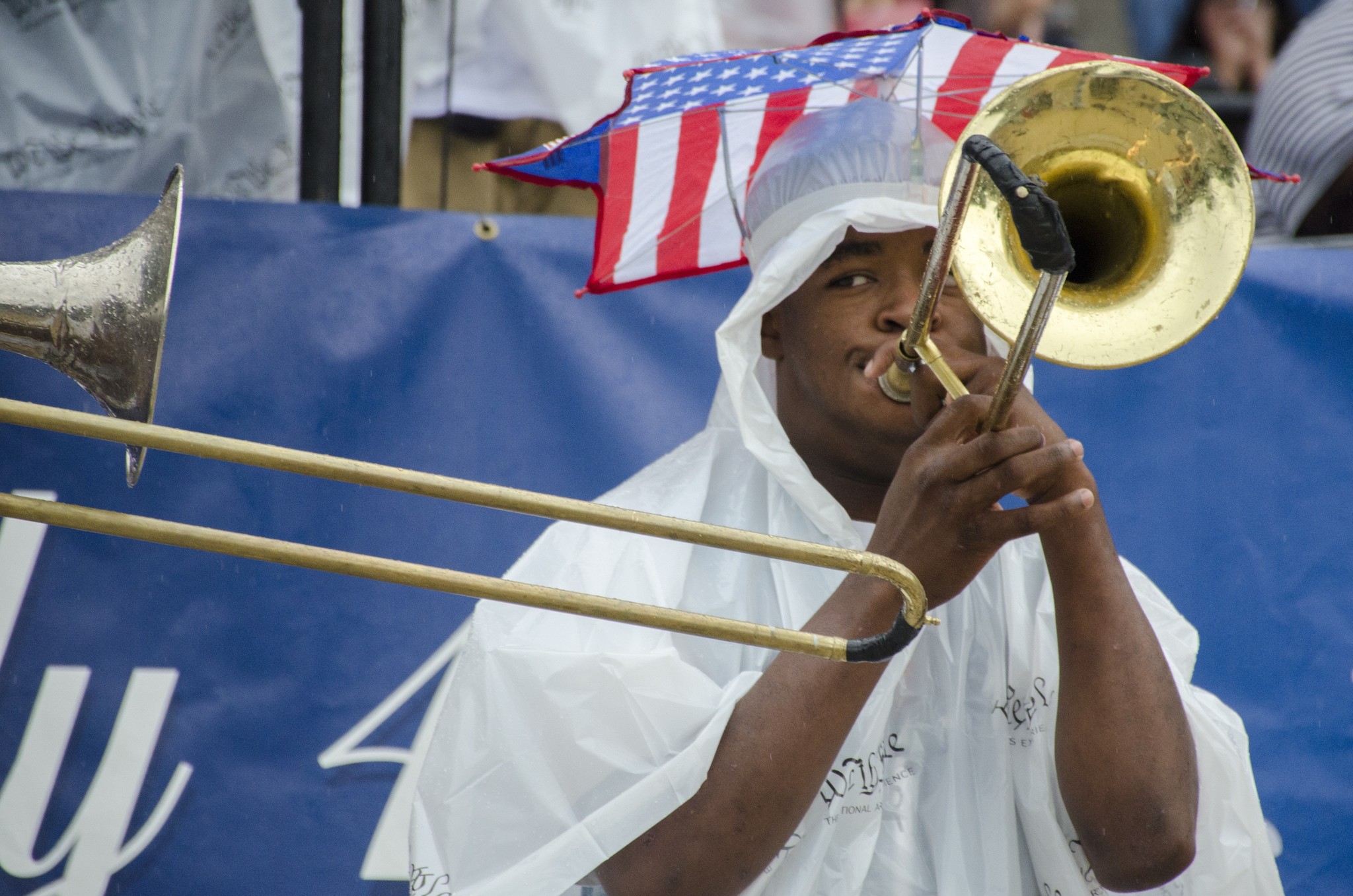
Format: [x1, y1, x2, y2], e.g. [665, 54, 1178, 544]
[404, 98, 1287, 896]
[387, 2, 1347, 243]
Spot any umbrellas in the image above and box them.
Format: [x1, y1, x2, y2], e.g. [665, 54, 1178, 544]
[471, 8, 1301, 298]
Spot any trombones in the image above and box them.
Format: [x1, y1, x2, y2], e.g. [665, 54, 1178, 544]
[878, 61, 1256, 430]
[0, 158, 924, 661]
